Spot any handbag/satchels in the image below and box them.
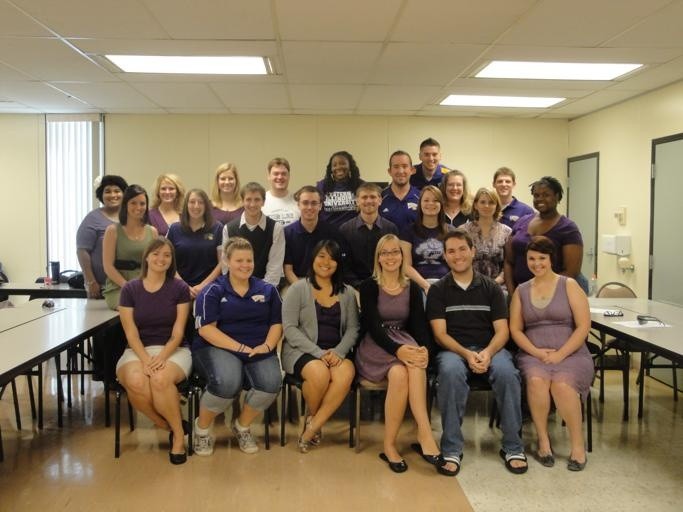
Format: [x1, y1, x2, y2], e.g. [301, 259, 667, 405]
[66, 272, 84, 288]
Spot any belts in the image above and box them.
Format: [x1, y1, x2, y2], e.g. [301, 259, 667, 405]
[382, 323, 403, 333]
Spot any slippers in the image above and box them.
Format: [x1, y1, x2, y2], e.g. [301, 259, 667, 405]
[411, 441, 463, 475]
[379, 453, 407, 472]
[499, 449, 528, 474]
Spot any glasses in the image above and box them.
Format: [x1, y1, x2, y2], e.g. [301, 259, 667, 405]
[603, 310, 622, 317]
[380, 250, 401, 257]
[637, 314, 665, 327]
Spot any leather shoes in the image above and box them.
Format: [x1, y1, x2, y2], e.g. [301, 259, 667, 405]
[567, 448, 585, 471]
[539, 435, 554, 467]
[169, 417, 189, 464]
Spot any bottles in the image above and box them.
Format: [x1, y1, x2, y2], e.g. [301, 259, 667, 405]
[51, 262, 59, 285]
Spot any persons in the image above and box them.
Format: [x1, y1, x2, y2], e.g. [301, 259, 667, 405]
[354, 234, 447, 473]
[116, 237, 196, 466]
[509, 236, 595, 471]
[188, 236, 284, 457]
[75, 136, 589, 395]
[423, 232, 528, 474]
[280, 240, 361, 454]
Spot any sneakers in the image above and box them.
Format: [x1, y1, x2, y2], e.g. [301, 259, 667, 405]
[231, 417, 259, 454]
[193, 416, 215, 459]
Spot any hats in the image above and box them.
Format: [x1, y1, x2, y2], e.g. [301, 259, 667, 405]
[94, 175, 128, 203]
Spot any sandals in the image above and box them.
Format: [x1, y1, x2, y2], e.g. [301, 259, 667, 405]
[299, 416, 322, 452]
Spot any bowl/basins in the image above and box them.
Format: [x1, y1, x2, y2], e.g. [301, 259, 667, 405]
[8, 295, 30, 308]
